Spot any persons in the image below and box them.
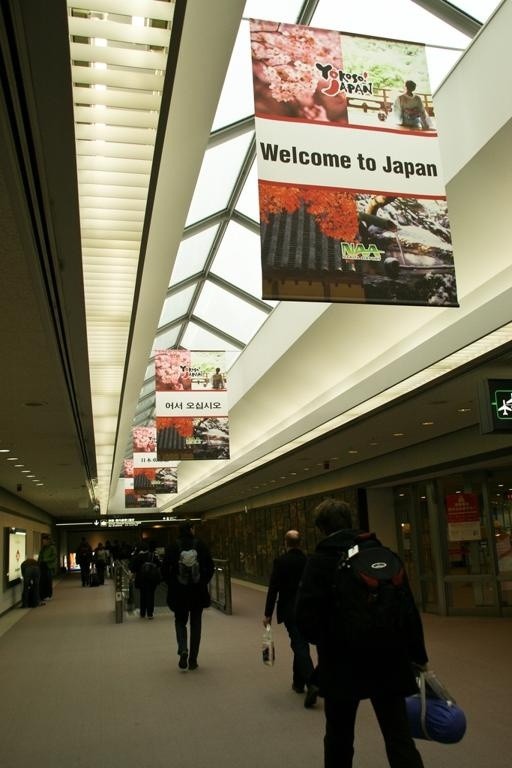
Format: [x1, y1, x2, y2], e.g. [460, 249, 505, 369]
[394, 80, 429, 130]
[263, 530, 318, 708]
[128, 542, 161, 620]
[164, 518, 215, 671]
[37, 535, 58, 601]
[76, 538, 132, 587]
[212, 368, 224, 389]
[290, 498, 424, 768]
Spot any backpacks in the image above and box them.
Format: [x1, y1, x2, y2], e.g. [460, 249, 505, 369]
[307, 532, 409, 658]
[176, 538, 201, 585]
[139, 554, 160, 583]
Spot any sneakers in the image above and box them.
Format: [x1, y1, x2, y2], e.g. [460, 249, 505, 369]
[178, 653, 188, 671]
[187, 663, 200, 672]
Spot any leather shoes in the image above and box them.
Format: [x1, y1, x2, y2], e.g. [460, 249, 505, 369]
[291, 682, 306, 694]
[303, 682, 320, 708]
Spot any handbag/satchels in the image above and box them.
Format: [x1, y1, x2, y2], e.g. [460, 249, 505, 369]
[402, 667, 466, 746]
